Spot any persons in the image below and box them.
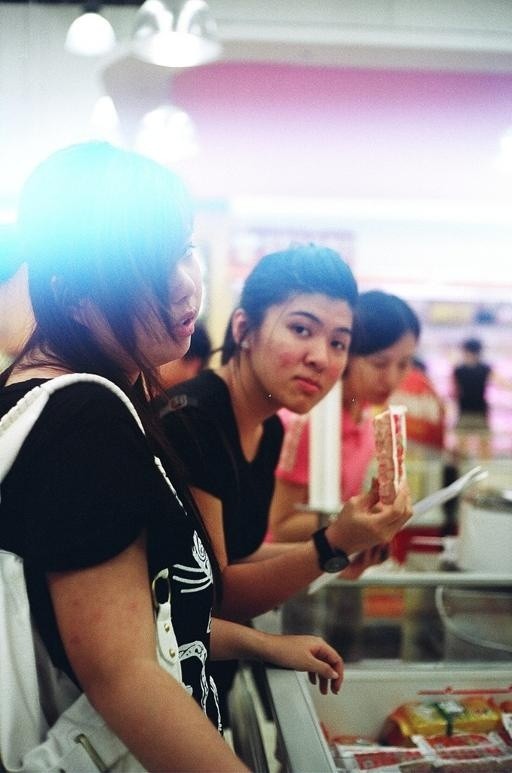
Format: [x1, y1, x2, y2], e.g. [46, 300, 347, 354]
[0, 138, 345, 773]
[0, 235, 493, 710]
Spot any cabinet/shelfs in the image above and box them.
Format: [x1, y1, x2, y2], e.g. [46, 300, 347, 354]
[227, 571, 512, 773]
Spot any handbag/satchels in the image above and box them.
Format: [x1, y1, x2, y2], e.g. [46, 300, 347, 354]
[0, 554, 183, 771]
